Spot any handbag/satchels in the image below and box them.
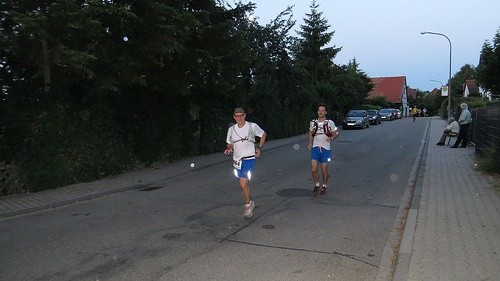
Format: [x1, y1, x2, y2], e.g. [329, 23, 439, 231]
[444, 129, 451, 134]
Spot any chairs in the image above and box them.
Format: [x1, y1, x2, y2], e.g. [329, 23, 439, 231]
[447, 132, 459, 146]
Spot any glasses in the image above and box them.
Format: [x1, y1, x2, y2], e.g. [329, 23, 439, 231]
[234, 114, 244, 118]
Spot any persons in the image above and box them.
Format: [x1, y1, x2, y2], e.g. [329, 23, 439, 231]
[308, 103, 340, 194]
[410, 105, 428, 123]
[223, 108, 267, 218]
[436, 117, 460, 146]
[449, 102, 472, 148]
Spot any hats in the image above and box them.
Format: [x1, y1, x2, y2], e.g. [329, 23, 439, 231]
[448, 117, 455, 121]
[234, 107, 244, 113]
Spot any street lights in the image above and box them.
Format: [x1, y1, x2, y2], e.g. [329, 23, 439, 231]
[421, 31, 452, 119]
[430, 80, 443, 119]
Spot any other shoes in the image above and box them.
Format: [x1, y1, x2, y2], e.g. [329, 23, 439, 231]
[450, 144, 458, 148]
[459, 144, 466, 148]
[240, 200, 255, 219]
[318, 186, 327, 195]
[436, 142, 445, 146]
[312, 185, 321, 196]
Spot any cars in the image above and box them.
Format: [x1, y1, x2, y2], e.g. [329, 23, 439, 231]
[342, 109, 370, 130]
[366, 109, 382, 125]
[379, 108, 393, 121]
[388, 108, 398, 120]
[394, 109, 402, 119]
